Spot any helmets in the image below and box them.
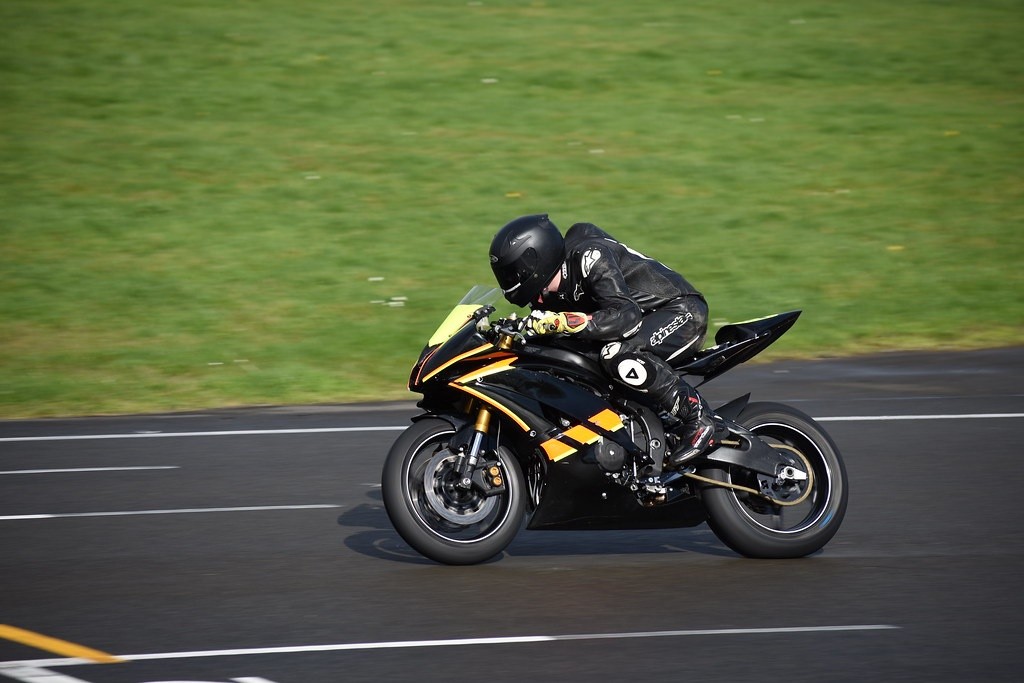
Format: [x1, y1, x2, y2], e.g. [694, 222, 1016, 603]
[490, 213, 564, 308]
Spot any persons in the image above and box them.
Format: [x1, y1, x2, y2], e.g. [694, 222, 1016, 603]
[489, 213, 730, 465]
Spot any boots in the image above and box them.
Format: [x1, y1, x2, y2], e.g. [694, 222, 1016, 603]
[658, 374, 730, 465]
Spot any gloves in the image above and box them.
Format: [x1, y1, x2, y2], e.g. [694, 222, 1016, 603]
[526, 309, 567, 335]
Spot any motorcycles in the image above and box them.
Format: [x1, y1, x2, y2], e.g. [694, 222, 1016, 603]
[381, 285, 850, 569]
